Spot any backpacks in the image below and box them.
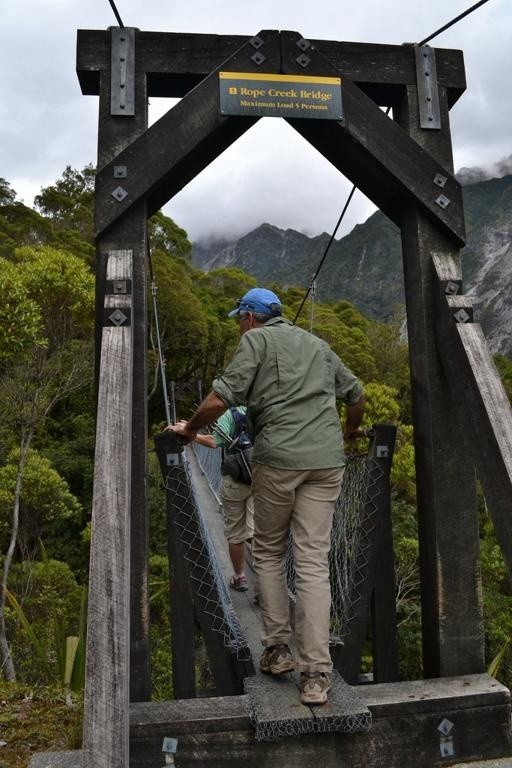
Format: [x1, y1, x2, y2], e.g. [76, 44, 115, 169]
[220, 406, 253, 486]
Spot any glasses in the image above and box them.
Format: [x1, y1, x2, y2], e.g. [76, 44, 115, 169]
[235, 298, 255, 309]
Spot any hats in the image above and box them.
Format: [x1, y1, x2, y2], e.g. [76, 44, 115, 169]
[227, 287, 283, 320]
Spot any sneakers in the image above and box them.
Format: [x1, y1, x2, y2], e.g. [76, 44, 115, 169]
[229, 574, 249, 591]
[300, 670, 332, 704]
[259, 643, 295, 674]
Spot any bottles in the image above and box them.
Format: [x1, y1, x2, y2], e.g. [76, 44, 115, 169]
[239, 442, 249, 449]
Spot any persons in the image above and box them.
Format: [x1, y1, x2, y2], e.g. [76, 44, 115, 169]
[163, 288, 363, 705]
[175, 403, 260, 601]
